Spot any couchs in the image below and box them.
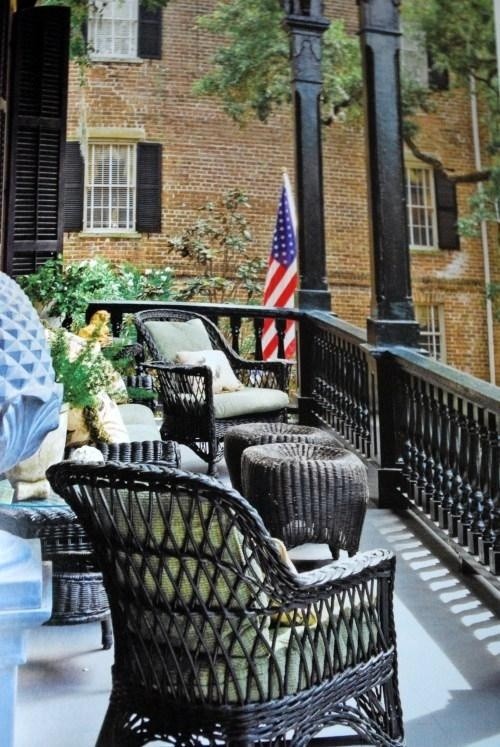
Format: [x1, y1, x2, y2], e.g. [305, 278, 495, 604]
[117, 400, 162, 444]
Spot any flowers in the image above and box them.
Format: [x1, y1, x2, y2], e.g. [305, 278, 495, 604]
[3, 310, 138, 408]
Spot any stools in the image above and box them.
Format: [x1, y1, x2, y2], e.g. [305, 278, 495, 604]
[223, 421, 369, 560]
[0, 500, 114, 651]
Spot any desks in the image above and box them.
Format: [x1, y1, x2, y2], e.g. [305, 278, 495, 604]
[62, 439, 181, 469]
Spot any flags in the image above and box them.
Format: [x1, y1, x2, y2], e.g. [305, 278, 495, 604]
[247, 169, 300, 387]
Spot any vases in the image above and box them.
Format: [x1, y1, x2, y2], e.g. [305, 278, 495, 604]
[5, 404, 71, 501]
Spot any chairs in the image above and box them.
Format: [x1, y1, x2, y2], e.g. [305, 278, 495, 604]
[45, 459, 500, 747]
[131, 308, 297, 479]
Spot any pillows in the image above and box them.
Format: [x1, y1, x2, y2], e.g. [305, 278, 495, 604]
[238, 533, 314, 626]
[67, 334, 129, 445]
[174, 348, 245, 396]
